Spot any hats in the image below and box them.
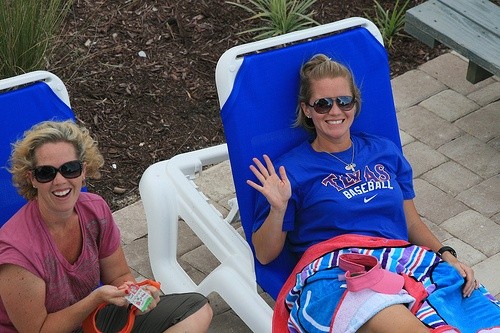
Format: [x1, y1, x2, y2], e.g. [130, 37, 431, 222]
[81, 279, 160, 333]
[339, 253, 404, 294]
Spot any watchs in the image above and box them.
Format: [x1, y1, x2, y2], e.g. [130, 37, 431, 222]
[436, 246, 457, 259]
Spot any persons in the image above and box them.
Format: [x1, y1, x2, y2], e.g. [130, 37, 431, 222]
[246, 54, 479, 333]
[1, 120, 213, 332]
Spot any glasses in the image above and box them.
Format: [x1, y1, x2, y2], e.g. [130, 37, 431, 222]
[306, 96, 356, 114]
[29, 160, 83, 183]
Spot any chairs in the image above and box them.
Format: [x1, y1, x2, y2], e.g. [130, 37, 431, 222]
[0, 70, 104, 287]
[139, 17, 404, 333]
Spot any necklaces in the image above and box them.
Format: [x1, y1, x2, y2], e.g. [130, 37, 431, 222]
[313, 139, 356, 172]
